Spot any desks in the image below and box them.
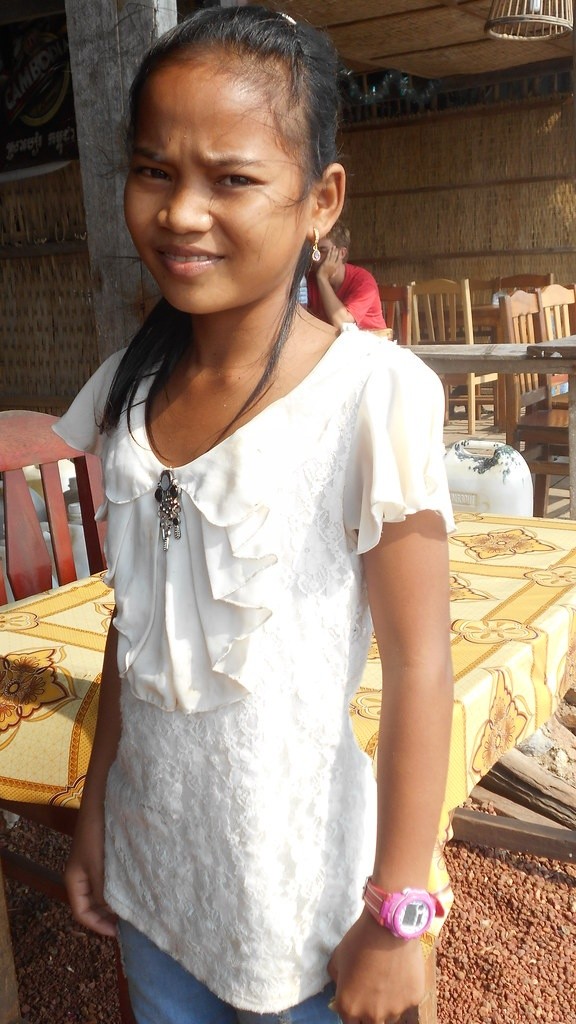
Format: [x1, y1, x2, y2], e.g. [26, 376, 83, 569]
[0, 514, 576, 1023]
[399, 343, 576, 436]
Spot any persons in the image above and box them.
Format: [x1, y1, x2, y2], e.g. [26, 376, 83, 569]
[307, 217, 388, 331]
[53, 5, 456, 1024]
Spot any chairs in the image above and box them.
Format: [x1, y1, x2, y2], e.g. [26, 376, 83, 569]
[0, 408, 110, 607]
[379, 272, 576, 519]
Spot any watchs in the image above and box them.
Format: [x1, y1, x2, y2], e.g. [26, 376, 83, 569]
[361, 875, 438, 943]
